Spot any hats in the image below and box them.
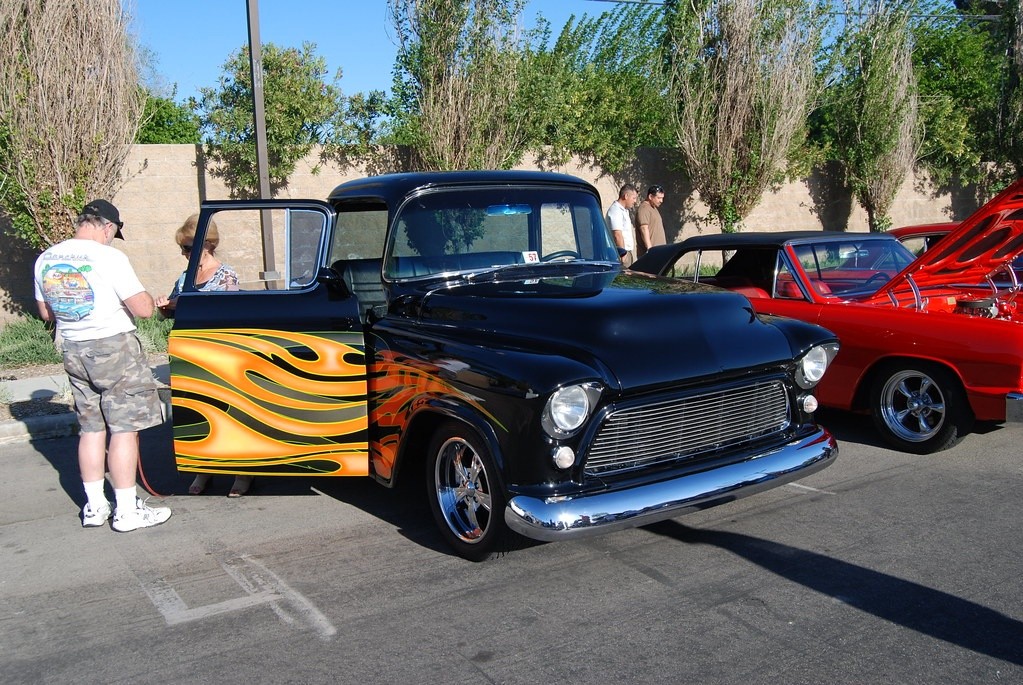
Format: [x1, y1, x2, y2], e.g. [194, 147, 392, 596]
[79, 199, 125, 241]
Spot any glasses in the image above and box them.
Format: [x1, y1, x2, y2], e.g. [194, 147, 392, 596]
[180, 240, 211, 253]
[106, 222, 123, 229]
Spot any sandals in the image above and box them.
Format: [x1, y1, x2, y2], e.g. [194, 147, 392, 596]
[228, 475, 253, 498]
[188, 473, 213, 495]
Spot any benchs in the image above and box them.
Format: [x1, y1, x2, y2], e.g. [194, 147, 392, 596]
[334, 249, 529, 323]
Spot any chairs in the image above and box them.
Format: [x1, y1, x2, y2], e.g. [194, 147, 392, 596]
[731, 287, 771, 300]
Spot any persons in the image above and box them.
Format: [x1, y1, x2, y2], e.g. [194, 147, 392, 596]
[34, 199, 171, 533]
[635, 185, 666, 259]
[605, 184, 638, 268]
[155, 214, 256, 498]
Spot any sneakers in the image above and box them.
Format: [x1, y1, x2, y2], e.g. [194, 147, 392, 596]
[82, 498, 117, 527]
[112, 496, 171, 532]
